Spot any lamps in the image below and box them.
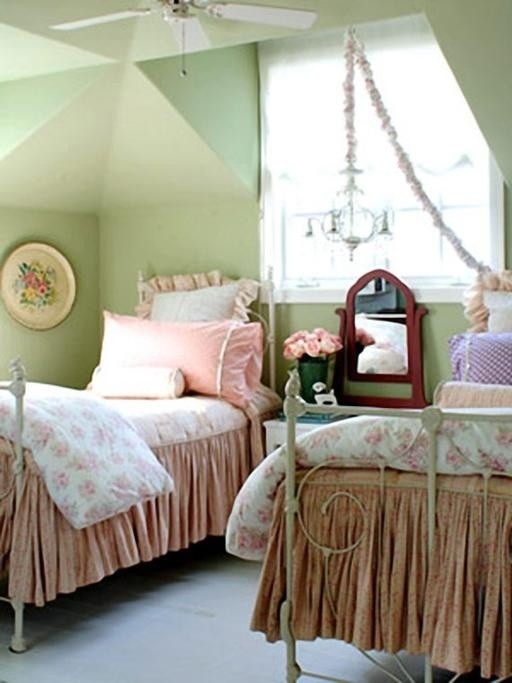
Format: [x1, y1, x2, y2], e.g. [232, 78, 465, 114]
[304, 27, 491, 273]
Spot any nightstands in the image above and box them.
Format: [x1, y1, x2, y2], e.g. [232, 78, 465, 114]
[263, 416, 331, 458]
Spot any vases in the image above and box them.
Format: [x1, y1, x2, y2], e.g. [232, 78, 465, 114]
[297, 361, 329, 413]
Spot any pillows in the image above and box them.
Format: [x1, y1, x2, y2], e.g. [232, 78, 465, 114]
[433, 275, 512, 409]
[92, 270, 265, 411]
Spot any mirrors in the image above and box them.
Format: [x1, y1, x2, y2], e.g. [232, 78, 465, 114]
[334, 269, 428, 408]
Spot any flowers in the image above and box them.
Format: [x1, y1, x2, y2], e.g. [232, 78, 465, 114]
[355, 325, 375, 351]
[285, 325, 343, 359]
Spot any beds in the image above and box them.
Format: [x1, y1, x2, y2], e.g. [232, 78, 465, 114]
[280, 370, 512, 683]
[0, 264, 277, 655]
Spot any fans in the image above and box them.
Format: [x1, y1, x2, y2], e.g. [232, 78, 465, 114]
[50, 0, 317, 79]
